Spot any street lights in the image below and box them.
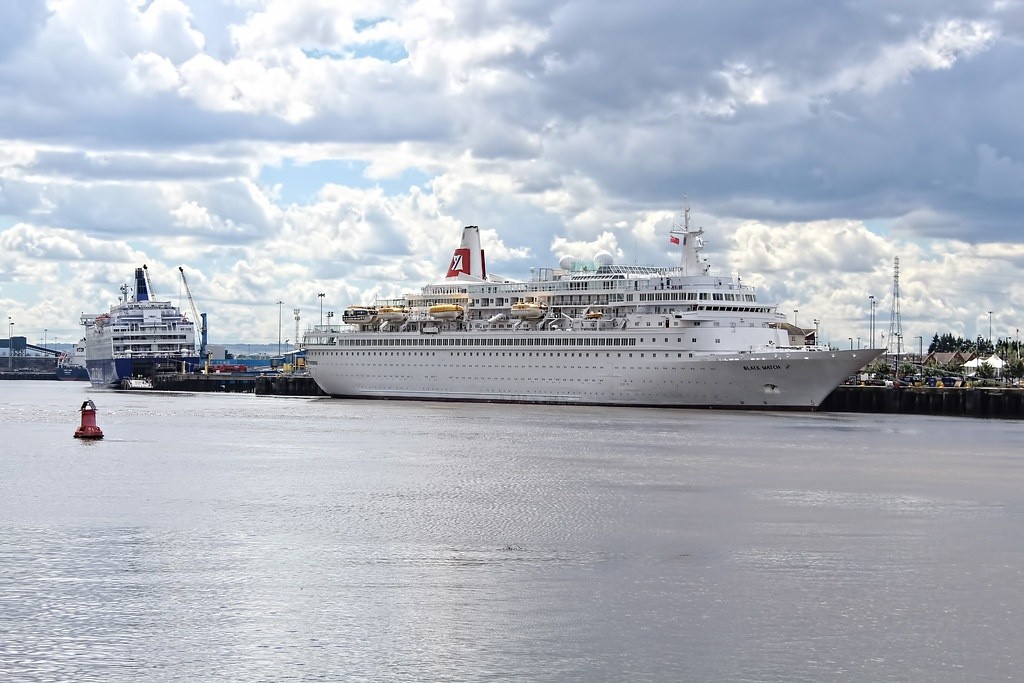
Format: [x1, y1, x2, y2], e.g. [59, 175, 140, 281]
[898, 336, 922, 361]
[277, 293, 325, 357]
[869, 296, 875, 349]
[794, 310, 798, 327]
[849, 338, 853, 351]
[813, 319, 820, 346]
[857, 337, 860, 349]
[8, 316, 48, 367]
[989, 311, 1011, 358]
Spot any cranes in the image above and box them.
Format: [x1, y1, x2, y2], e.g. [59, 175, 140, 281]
[143, 264, 203, 348]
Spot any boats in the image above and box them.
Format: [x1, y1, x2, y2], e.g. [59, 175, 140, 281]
[56, 268, 199, 389]
[429, 303, 462, 318]
[293, 193, 890, 411]
[580, 311, 603, 319]
[511, 301, 549, 318]
[342, 306, 379, 325]
[377, 305, 413, 322]
